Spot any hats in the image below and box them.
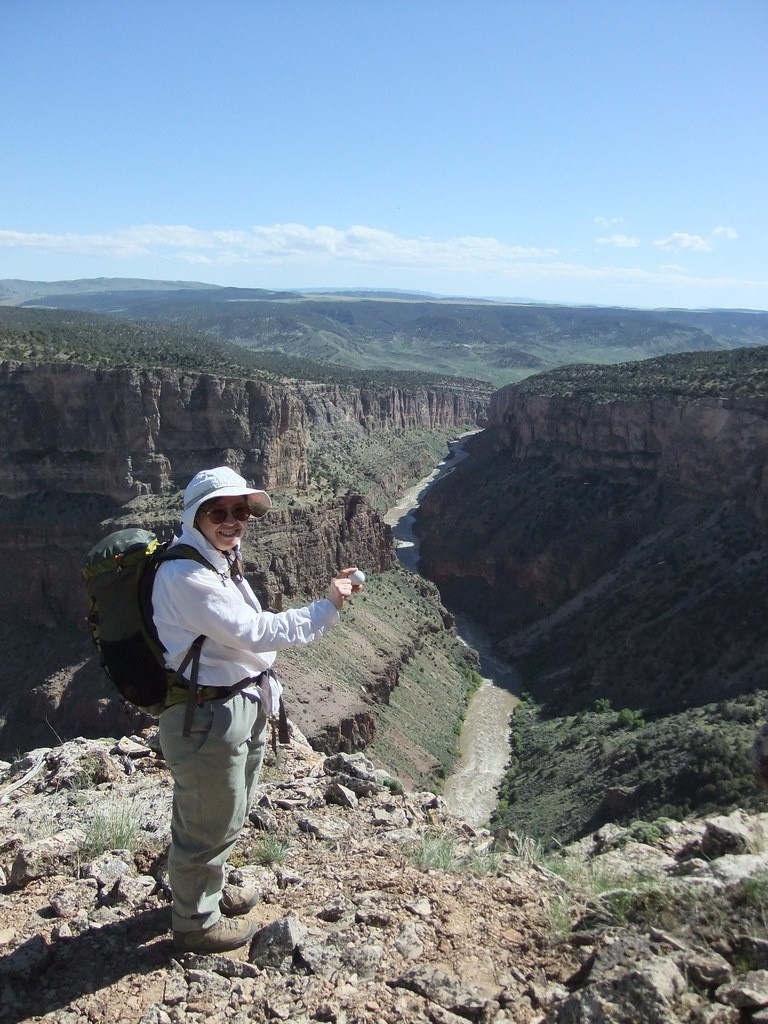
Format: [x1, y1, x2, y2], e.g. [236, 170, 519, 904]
[180, 466, 272, 531]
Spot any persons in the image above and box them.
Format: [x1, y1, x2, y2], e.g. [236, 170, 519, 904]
[151, 466, 362, 953]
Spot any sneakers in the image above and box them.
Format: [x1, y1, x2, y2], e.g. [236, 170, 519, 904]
[173, 914, 257, 952]
[218, 883, 258, 916]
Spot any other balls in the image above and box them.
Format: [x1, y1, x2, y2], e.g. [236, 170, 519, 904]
[348, 569, 366, 585]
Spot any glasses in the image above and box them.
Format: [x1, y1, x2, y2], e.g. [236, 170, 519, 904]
[198, 505, 254, 524]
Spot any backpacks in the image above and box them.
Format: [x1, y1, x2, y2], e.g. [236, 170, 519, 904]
[80, 527, 224, 718]
